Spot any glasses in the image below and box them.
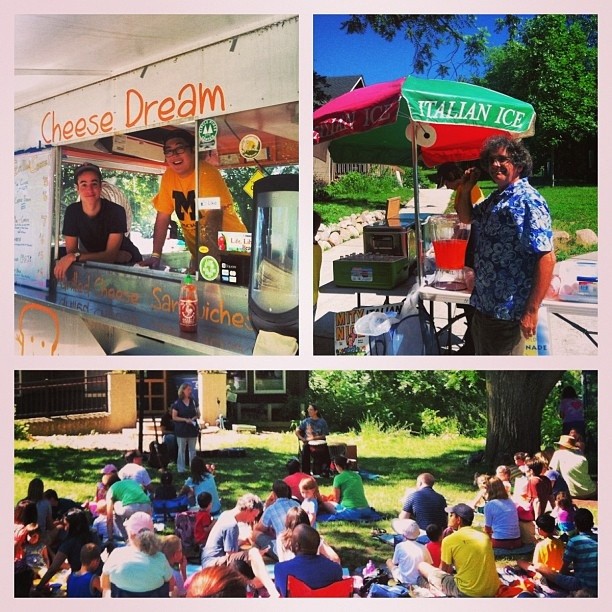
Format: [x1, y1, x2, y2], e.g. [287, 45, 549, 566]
[166, 145, 190, 158]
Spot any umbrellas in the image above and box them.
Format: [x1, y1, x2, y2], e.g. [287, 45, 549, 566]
[313, 73, 539, 170]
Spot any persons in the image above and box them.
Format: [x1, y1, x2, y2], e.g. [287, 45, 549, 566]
[64, 542, 104, 597]
[118, 448, 153, 490]
[99, 511, 176, 597]
[562, 508, 598, 595]
[317, 456, 384, 522]
[341, 334, 360, 355]
[392, 473, 450, 538]
[263, 458, 317, 509]
[45, 489, 80, 519]
[94, 464, 117, 500]
[384, 516, 434, 585]
[14, 563, 35, 598]
[298, 474, 320, 529]
[251, 479, 301, 562]
[549, 435, 597, 500]
[191, 492, 216, 546]
[54, 163, 139, 281]
[296, 404, 329, 478]
[159, 533, 189, 599]
[173, 512, 192, 542]
[160, 401, 177, 459]
[558, 384, 587, 440]
[422, 522, 445, 567]
[273, 522, 347, 598]
[473, 448, 579, 550]
[275, 502, 343, 563]
[152, 472, 188, 512]
[533, 508, 598, 598]
[313, 207, 323, 317]
[183, 566, 247, 598]
[91, 498, 119, 541]
[436, 158, 483, 207]
[418, 503, 503, 596]
[35, 511, 97, 595]
[567, 427, 583, 451]
[455, 134, 557, 356]
[13, 498, 37, 560]
[21, 521, 51, 578]
[138, 128, 248, 271]
[107, 474, 153, 539]
[181, 457, 221, 515]
[14, 475, 53, 521]
[171, 382, 200, 474]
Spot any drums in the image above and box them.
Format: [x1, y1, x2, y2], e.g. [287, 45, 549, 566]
[308, 440, 326, 475]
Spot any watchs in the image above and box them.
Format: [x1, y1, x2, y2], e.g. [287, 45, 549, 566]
[73, 248, 82, 263]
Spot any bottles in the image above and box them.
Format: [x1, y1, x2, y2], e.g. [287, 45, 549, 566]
[178, 275, 199, 333]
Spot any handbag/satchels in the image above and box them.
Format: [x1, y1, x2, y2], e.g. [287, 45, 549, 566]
[152, 493, 189, 522]
[358, 567, 389, 596]
[369, 297, 437, 355]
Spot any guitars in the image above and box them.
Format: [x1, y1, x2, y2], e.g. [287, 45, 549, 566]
[148, 416, 168, 467]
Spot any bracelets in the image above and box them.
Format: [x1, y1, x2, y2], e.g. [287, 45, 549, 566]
[150, 250, 162, 259]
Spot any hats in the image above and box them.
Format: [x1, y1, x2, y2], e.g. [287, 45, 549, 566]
[437, 161, 460, 188]
[122, 511, 153, 535]
[515, 501, 533, 521]
[103, 464, 116, 474]
[391, 518, 419, 539]
[74, 162, 101, 178]
[444, 503, 474, 522]
[553, 434, 580, 449]
